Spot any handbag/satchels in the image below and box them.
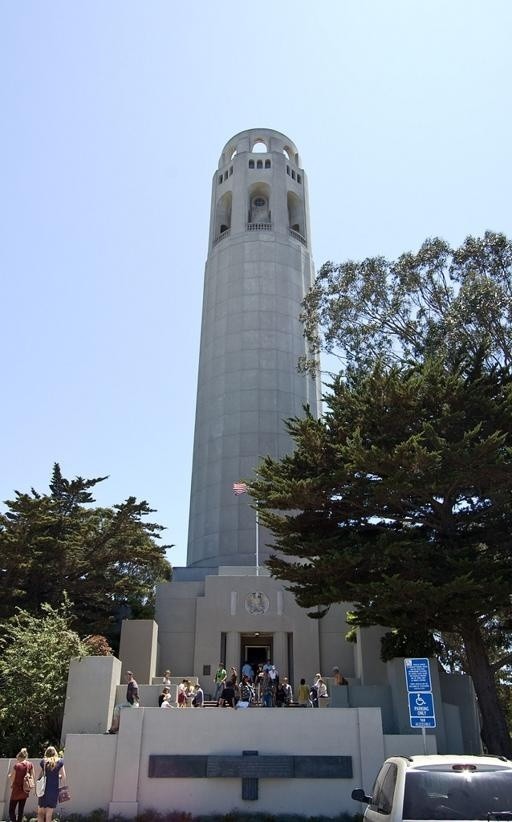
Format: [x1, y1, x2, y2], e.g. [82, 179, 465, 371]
[236, 701, 248, 708]
[59, 785, 71, 802]
[218, 698, 224, 706]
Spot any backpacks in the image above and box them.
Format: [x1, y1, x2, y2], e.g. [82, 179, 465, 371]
[23, 763, 35, 793]
[36, 776, 47, 797]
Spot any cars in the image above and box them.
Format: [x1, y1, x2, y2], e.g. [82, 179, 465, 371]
[352, 755, 512, 822]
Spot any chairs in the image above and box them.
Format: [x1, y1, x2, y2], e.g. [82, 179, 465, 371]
[404, 787, 511, 820]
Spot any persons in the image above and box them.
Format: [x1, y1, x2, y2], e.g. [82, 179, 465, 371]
[8, 747, 34, 822]
[37, 746, 66, 822]
[103, 670, 139, 734]
[158, 657, 348, 708]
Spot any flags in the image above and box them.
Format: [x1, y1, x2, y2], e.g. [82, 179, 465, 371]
[233, 481, 251, 495]
